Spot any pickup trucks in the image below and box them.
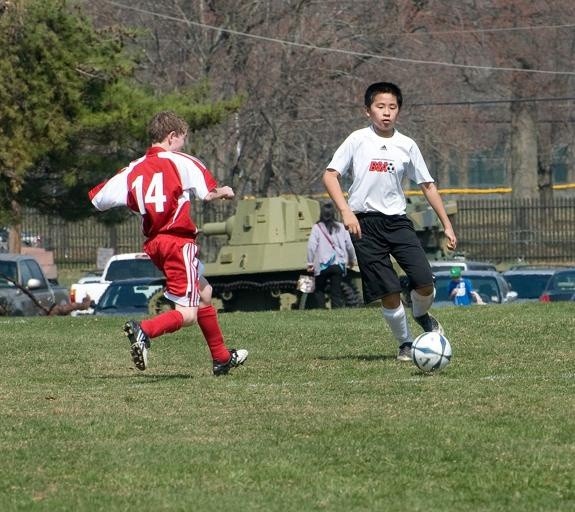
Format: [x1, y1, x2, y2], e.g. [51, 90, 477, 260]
[69, 252, 167, 316]
[0, 254, 71, 317]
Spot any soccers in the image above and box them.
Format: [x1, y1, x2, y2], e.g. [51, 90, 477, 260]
[386, 163, 395, 173]
[411, 332, 452, 372]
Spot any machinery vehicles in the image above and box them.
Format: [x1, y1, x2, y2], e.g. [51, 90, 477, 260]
[147, 196, 414, 313]
[390, 194, 462, 275]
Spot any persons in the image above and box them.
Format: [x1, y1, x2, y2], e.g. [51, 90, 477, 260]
[87, 111, 249, 375]
[306, 204, 357, 309]
[321, 81, 457, 362]
[449, 266, 484, 307]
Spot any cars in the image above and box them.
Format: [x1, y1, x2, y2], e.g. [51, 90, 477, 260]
[89, 278, 172, 317]
[423, 262, 575, 308]
[0, 227, 44, 250]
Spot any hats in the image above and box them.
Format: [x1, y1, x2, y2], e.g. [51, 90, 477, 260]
[450, 267, 461, 278]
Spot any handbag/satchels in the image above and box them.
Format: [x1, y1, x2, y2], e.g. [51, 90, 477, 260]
[338, 259, 348, 277]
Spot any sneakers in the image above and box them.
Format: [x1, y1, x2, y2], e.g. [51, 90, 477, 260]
[397, 342, 413, 361]
[122, 320, 151, 371]
[213, 349, 249, 375]
[413, 312, 444, 336]
